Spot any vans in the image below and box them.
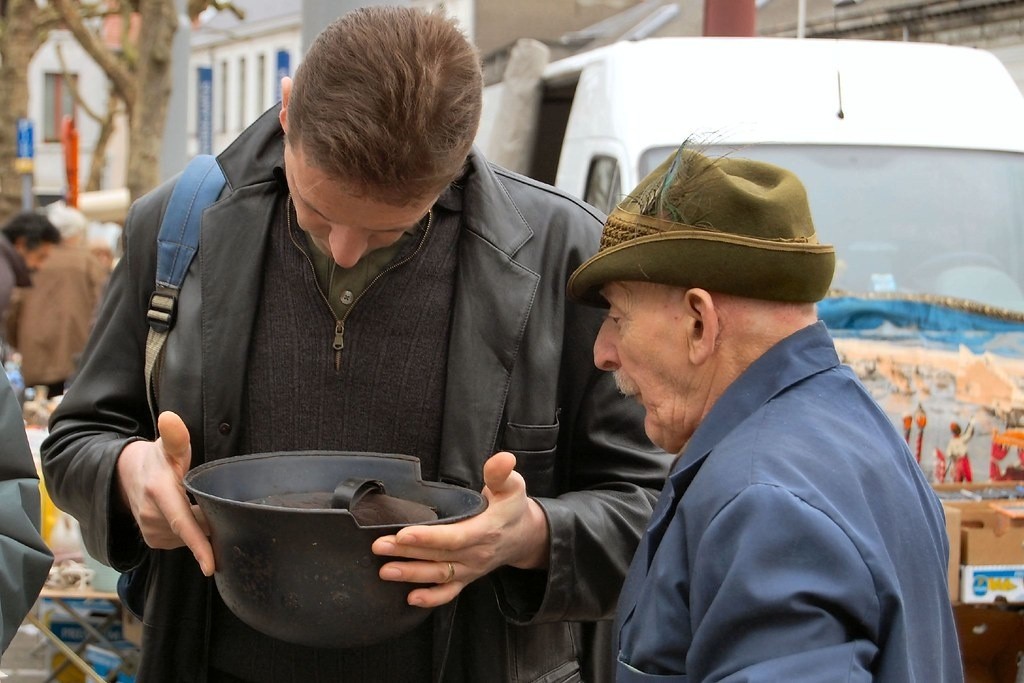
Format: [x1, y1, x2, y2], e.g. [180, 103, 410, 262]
[469, 34, 1024, 683]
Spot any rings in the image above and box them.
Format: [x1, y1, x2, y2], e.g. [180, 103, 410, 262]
[445, 563, 455, 583]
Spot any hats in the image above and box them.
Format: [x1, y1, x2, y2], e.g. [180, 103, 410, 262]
[567, 149, 836, 302]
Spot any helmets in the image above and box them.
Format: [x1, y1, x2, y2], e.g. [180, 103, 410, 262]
[180, 451, 487, 647]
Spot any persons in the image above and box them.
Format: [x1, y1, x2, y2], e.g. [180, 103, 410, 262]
[569, 132, 963, 683]
[0, 361, 54, 658]
[948, 422, 974, 482]
[0, 201, 115, 402]
[41, 6, 677, 683]
